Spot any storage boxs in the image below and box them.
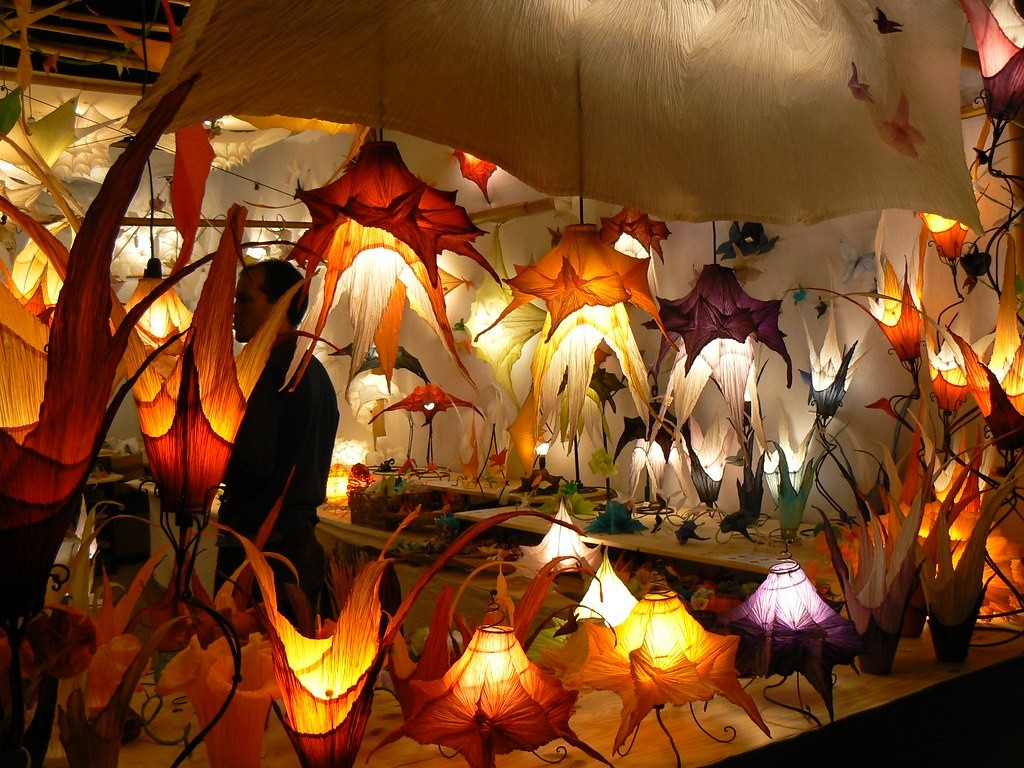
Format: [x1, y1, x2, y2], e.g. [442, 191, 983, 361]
[348, 487, 438, 532]
[112, 450, 145, 496]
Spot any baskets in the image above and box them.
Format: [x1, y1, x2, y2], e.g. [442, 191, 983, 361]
[349, 489, 444, 531]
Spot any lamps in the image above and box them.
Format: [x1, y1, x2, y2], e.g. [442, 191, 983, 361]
[0, 0, 1024, 768]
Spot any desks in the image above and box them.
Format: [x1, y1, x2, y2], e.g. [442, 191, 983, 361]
[452, 500, 841, 593]
[370, 454, 618, 506]
[27, 614, 1024, 768]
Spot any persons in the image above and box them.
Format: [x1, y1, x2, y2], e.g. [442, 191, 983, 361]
[212, 258, 340, 617]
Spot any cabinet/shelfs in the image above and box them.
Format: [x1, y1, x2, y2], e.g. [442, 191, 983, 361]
[126, 481, 227, 605]
